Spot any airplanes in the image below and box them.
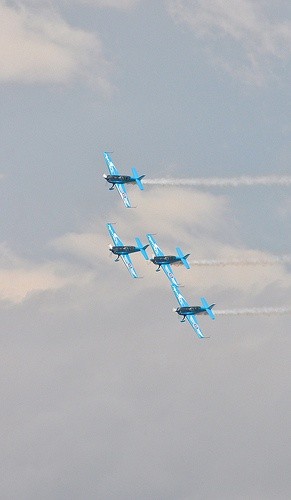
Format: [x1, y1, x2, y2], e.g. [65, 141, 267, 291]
[147, 233, 193, 288]
[171, 284, 215, 339]
[105, 222, 149, 278]
[102, 150, 147, 209]
